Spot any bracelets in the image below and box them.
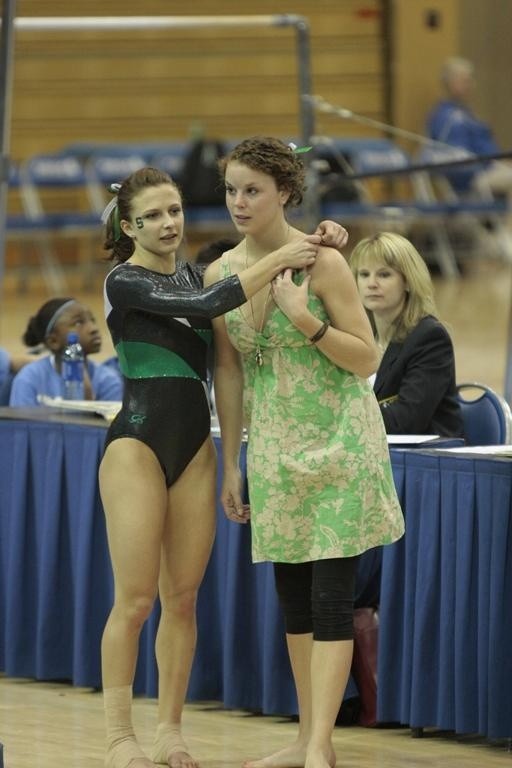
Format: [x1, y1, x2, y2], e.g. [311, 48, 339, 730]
[310, 322, 330, 341]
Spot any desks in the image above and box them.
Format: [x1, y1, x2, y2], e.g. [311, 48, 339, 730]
[0, 403, 511, 742]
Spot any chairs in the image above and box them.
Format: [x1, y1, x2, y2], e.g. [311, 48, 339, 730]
[447, 379, 511, 449]
[1, 136, 512, 300]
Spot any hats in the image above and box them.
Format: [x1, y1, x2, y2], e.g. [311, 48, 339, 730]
[36, 296, 74, 341]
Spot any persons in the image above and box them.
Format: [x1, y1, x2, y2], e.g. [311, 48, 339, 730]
[203, 135, 406, 767]
[347, 231, 465, 439]
[8, 296, 124, 405]
[0, 350, 44, 405]
[97, 166, 351, 767]
[429, 61, 510, 200]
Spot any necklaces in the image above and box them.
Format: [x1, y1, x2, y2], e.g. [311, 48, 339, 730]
[245, 224, 290, 366]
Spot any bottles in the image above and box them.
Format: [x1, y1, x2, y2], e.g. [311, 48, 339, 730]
[62, 333, 84, 399]
